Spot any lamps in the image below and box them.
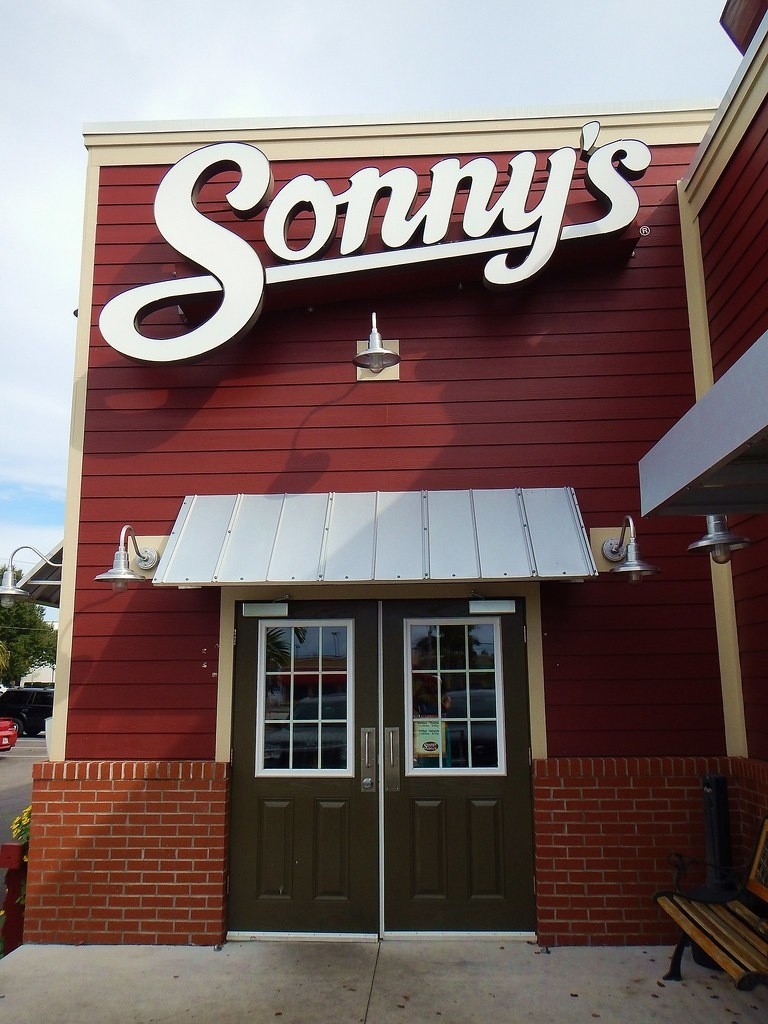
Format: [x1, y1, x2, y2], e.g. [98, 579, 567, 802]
[0, 545, 62, 608]
[93, 525, 170, 593]
[351, 312, 401, 381]
[686, 515, 754, 565]
[589, 515, 663, 582]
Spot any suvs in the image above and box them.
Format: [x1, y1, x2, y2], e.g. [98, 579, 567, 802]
[0, 688, 54, 736]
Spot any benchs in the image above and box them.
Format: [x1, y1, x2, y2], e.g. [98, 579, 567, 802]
[653, 817, 768, 993]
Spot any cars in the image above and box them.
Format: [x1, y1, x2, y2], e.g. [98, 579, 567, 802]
[270, 694, 347, 768]
[0, 717, 19, 751]
[437, 689, 498, 768]
